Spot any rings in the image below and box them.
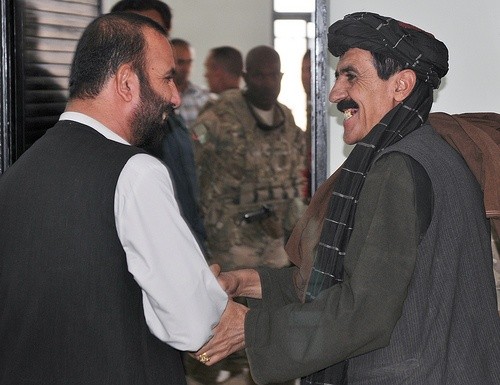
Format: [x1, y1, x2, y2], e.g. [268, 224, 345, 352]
[200, 352, 210, 363]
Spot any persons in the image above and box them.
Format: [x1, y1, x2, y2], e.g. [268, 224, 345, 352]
[189, 12, 500, 385]
[0, 11, 229, 385]
[110, 0, 312, 385]
